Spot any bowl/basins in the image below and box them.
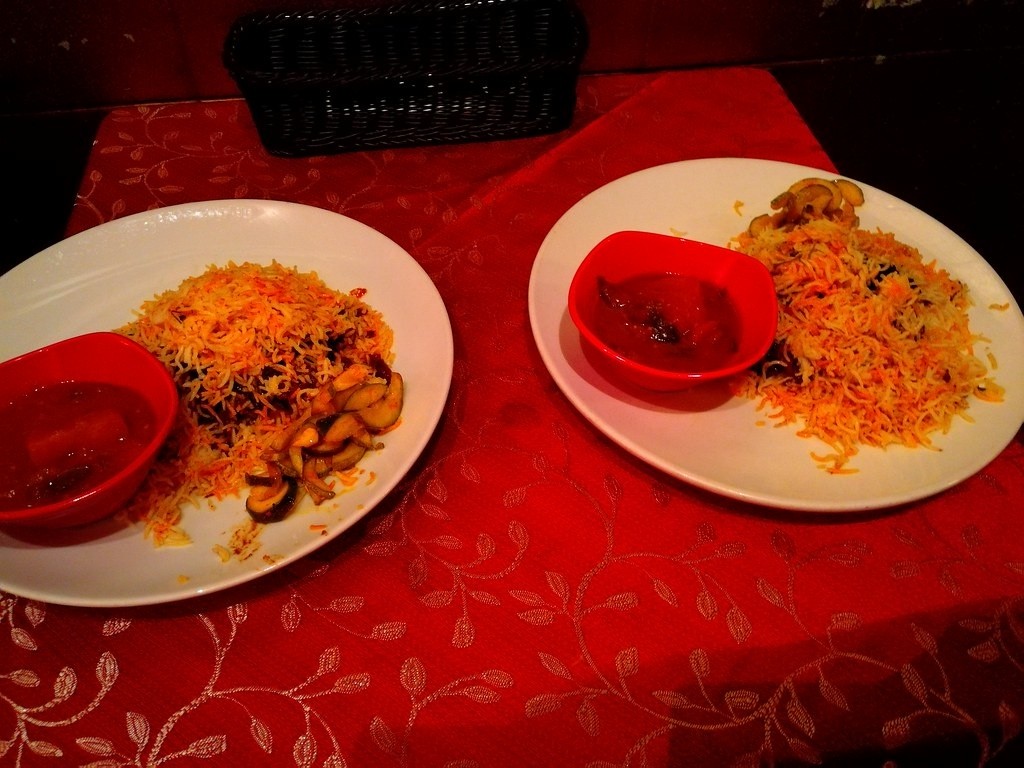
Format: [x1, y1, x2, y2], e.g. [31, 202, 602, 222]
[568, 230, 779, 393]
[0, 332, 179, 532]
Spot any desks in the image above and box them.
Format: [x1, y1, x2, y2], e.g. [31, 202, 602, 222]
[0, 48, 1024, 768]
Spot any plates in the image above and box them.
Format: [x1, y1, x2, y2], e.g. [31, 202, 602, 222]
[527, 158, 1024, 514]
[0, 198, 454, 609]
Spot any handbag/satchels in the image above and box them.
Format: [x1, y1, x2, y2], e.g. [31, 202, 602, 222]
[219, 0, 587, 158]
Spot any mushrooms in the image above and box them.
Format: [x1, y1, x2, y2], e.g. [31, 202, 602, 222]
[749, 177, 864, 238]
[245, 353, 403, 523]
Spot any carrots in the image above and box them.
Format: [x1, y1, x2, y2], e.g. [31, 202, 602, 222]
[759, 227, 998, 445]
[126, 265, 379, 534]
